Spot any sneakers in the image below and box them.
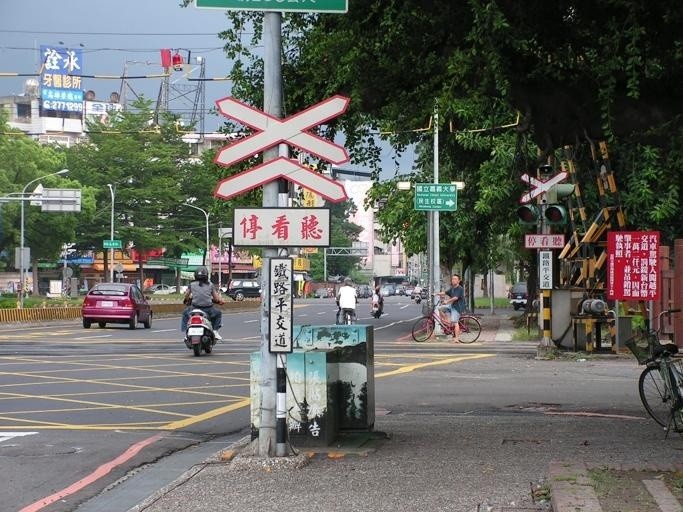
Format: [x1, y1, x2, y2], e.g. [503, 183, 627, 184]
[213, 330, 222, 340]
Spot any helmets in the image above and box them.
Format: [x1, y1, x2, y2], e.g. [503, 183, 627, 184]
[194, 266, 208, 276]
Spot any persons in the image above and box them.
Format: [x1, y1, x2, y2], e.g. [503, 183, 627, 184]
[370, 285, 383, 312]
[334, 276, 358, 324]
[430, 275, 464, 343]
[413, 283, 422, 295]
[179, 266, 223, 343]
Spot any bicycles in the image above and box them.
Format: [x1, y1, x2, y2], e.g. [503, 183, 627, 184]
[623, 307, 683, 442]
[333, 300, 358, 325]
[410, 294, 481, 343]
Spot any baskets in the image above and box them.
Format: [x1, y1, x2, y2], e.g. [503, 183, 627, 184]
[624, 329, 660, 366]
[422, 302, 434, 316]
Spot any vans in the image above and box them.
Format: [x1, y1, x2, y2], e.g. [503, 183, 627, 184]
[508, 281, 528, 310]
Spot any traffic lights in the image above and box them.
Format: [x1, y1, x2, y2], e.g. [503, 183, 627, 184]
[557, 184, 576, 200]
[540, 204, 568, 225]
[517, 203, 541, 224]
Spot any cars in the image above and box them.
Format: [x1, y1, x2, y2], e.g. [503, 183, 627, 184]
[80, 282, 153, 330]
[149, 283, 170, 294]
[314, 283, 428, 300]
[169, 286, 183, 293]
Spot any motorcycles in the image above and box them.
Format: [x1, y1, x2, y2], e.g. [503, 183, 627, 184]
[412, 290, 422, 304]
[368, 294, 384, 318]
[183, 297, 225, 356]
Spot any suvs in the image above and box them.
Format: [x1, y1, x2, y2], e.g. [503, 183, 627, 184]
[227, 278, 261, 300]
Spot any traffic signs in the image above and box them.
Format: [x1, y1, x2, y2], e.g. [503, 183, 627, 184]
[413, 183, 458, 212]
[195, 1, 348, 13]
[102, 240, 120, 249]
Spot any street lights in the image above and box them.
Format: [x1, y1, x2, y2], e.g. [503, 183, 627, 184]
[105, 183, 114, 282]
[181, 200, 231, 281]
[17, 169, 69, 308]
